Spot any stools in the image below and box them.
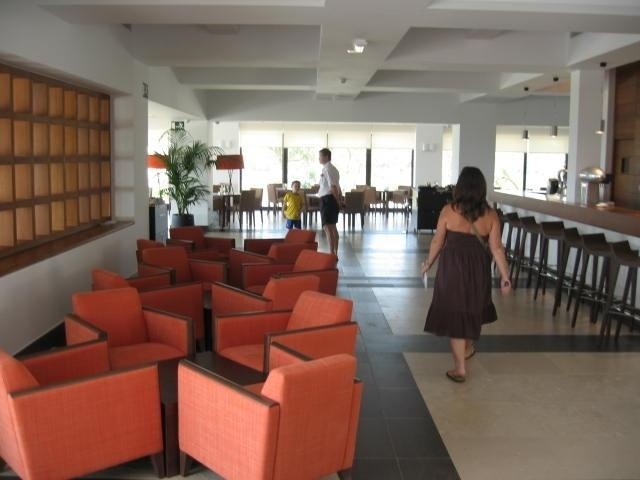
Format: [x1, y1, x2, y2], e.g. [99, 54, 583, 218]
[488, 207, 640, 354]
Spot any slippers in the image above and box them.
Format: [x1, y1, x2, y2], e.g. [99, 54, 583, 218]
[462, 342, 476, 360]
[445, 368, 467, 384]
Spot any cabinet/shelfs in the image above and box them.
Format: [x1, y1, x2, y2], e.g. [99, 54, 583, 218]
[416, 185, 455, 235]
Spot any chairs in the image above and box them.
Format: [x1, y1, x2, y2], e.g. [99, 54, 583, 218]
[212, 290, 358, 377]
[1, 337, 165, 479]
[179, 341, 362, 479]
[91, 225, 339, 349]
[215, 182, 415, 230]
[64, 286, 194, 375]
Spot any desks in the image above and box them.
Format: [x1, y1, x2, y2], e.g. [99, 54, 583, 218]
[156, 344, 265, 477]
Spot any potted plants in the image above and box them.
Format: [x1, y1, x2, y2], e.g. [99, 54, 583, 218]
[149, 122, 227, 230]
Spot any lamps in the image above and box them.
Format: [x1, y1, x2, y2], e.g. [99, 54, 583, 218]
[348, 37, 368, 57]
[549, 75, 560, 138]
[214, 153, 244, 197]
[595, 59, 609, 134]
[423, 143, 434, 152]
[148, 155, 172, 196]
[521, 86, 531, 141]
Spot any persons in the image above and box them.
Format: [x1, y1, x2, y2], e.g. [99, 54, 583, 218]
[318, 147, 342, 261]
[282, 180, 305, 233]
[421, 166, 512, 384]
